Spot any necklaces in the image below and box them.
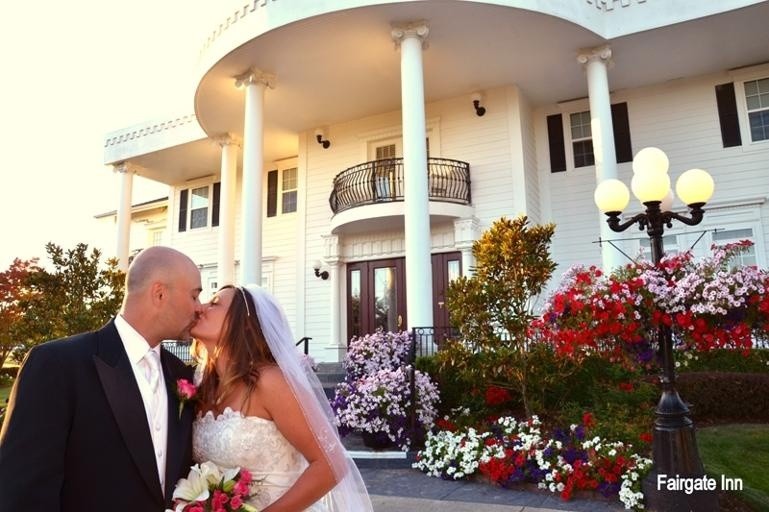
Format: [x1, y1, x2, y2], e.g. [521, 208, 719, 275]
[213, 375, 242, 408]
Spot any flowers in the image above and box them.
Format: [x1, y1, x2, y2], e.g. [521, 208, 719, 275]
[163, 459, 266, 512]
[165, 375, 205, 421]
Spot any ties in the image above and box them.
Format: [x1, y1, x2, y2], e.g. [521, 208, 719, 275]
[142, 350, 166, 487]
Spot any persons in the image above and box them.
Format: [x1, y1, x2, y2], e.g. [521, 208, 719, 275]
[0, 246, 203, 512]
[191, 281, 373, 512]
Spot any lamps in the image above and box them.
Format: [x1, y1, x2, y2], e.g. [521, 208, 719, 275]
[315, 127, 330, 151]
[470, 92, 488, 118]
[311, 259, 330, 279]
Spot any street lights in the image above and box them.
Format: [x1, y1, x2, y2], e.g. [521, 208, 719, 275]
[586, 143, 730, 511]
